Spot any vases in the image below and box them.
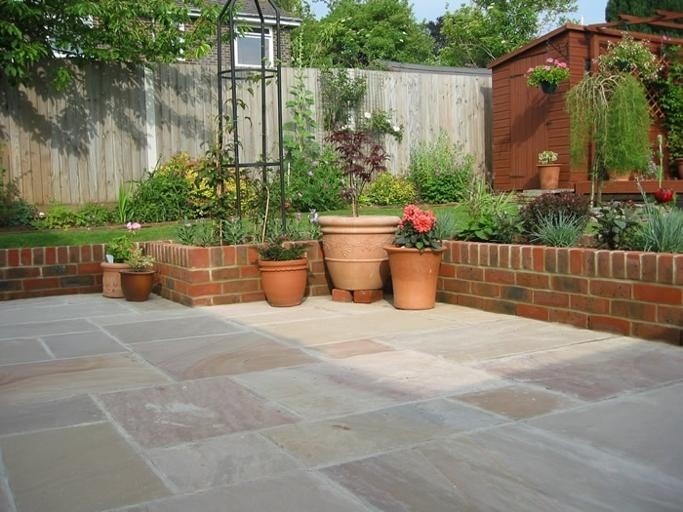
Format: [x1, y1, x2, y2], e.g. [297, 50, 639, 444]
[99, 261, 158, 303]
[381, 244, 448, 311]
[538, 79, 557, 92]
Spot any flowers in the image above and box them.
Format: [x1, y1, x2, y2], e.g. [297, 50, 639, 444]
[523, 56, 572, 88]
[104, 218, 160, 271]
[390, 202, 444, 251]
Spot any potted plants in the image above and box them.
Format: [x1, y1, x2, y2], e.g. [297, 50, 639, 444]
[534, 147, 562, 188]
[254, 122, 400, 308]
